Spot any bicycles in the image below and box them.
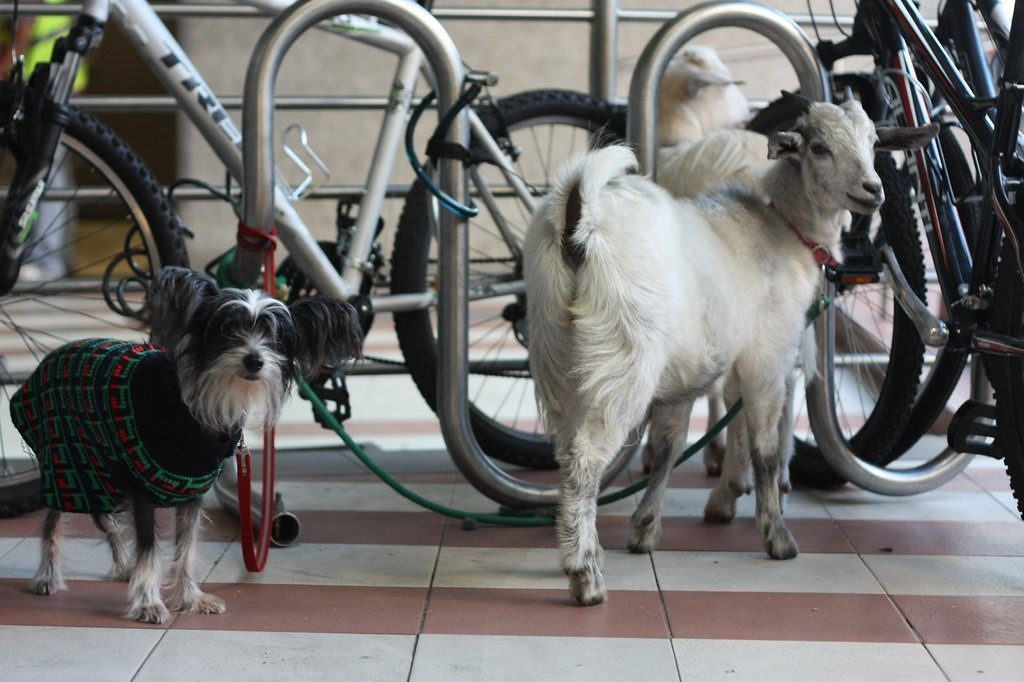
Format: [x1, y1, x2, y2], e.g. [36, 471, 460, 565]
[740, 1, 1024, 515]
[0, 2, 658, 524]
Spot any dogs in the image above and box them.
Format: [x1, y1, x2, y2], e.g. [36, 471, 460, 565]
[10, 266, 362, 625]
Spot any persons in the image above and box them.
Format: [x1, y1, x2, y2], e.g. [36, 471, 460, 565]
[0, 0, 88, 282]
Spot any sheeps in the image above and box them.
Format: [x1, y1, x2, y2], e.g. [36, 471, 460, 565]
[521, 46, 886, 605]
